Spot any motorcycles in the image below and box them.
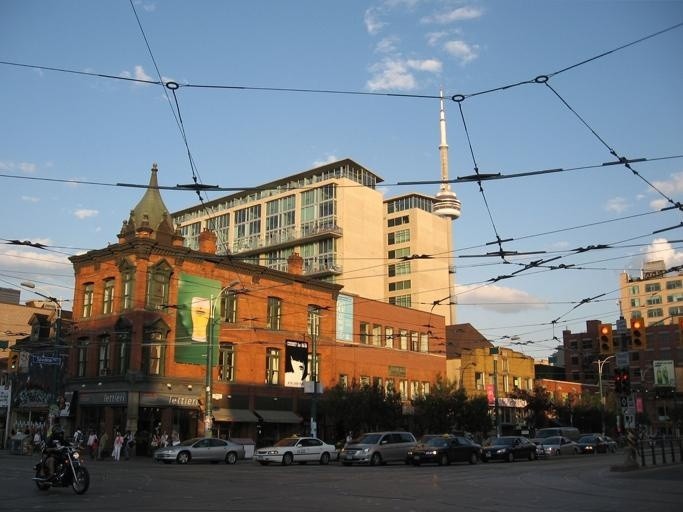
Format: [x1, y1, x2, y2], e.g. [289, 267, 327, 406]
[33, 439, 90, 494]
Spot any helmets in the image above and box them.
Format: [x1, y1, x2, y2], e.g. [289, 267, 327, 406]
[52, 424, 64, 435]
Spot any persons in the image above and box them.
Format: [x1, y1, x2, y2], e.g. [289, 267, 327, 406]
[626, 428, 637, 461]
[25, 421, 180, 480]
[346, 431, 353, 443]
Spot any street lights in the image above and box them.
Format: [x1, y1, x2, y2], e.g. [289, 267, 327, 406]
[493, 337, 522, 435]
[309, 315, 328, 435]
[598, 354, 616, 434]
[204, 280, 239, 439]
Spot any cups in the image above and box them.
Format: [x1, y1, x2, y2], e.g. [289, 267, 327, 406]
[191, 297, 210, 342]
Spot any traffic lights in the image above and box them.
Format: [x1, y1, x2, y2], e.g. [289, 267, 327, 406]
[597, 324, 614, 354]
[8, 352, 19, 373]
[630, 318, 647, 351]
[613, 366, 631, 393]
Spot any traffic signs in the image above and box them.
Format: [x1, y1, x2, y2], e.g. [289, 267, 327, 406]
[32, 355, 61, 365]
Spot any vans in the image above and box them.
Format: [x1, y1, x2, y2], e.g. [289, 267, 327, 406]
[338, 432, 417, 466]
[536, 427, 580, 440]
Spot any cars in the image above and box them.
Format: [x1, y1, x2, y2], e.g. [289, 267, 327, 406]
[155, 437, 245, 464]
[576, 435, 616, 454]
[253, 437, 338, 466]
[535, 436, 577, 457]
[480, 436, 537, 463]
[406, 436, 481, 466]
